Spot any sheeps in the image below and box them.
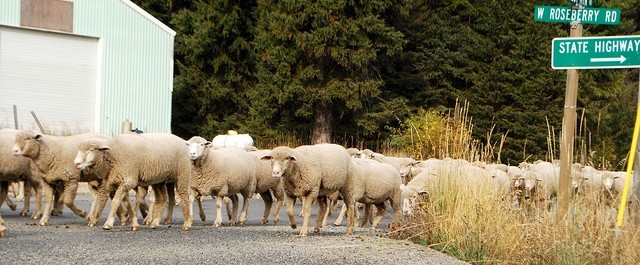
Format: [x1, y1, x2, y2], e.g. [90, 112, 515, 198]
[11, 129, 140, 231]
[0, 127, 44, 221]
[183, 134, 257, 231]
[260, 143, 355, 237]
[348, 148, 635, 237]
[247, 149, 284, 225]
[74, 132, 191, 231]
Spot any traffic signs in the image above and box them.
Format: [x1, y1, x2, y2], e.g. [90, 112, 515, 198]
[552, 36, 640, 69]
[535, 5, 621, 25]
